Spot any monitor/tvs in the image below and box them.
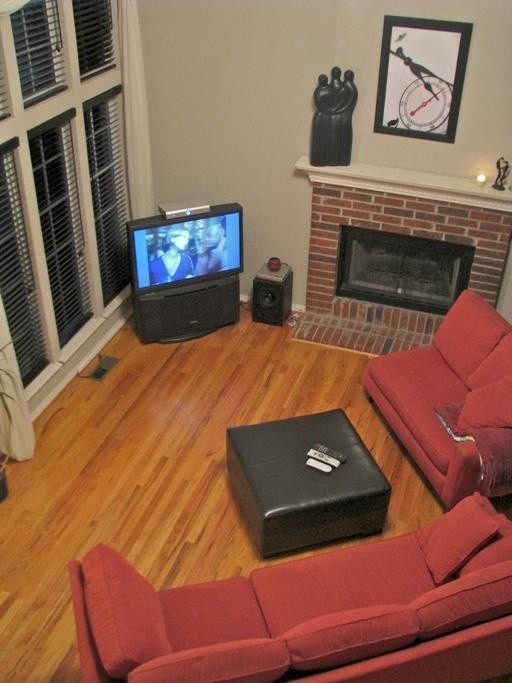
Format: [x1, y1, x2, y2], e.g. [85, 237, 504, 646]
[125, 202, 243, 297]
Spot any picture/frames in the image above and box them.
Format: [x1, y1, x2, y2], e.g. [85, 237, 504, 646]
[373, 15, 473, 143]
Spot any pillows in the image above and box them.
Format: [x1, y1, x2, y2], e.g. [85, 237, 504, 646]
[432, 290, 512, 381]
[415, 491, 502, 586]
[456, 372, 512, 430]
[276, 604, 420, 672]
[464, 330, 512, 391]
[127, 636, 292, 683]
[408, 559, 512, 638]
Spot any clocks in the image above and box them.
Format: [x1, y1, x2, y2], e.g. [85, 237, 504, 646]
[398, 75, 453, 132]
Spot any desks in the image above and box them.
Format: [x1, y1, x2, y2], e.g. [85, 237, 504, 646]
[226, 409, 392, 558]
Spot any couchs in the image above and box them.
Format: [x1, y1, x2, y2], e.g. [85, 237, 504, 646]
[68, 492, 512, 683]
[363, 290, 512, 511]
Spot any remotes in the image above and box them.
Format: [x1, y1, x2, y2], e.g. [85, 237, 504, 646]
[307, 448, 340, 469]
[312, 442, 347, 463]
[306, 458, 332, 472]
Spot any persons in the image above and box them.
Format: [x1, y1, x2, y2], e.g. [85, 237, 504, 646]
[149, 227, 194, 284]
[196, 222, 228, 276]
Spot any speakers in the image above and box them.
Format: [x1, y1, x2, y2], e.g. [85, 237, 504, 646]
[253, 268, 293, 326]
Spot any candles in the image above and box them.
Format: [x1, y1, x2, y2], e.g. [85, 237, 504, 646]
[476, 174, 486, 187]
